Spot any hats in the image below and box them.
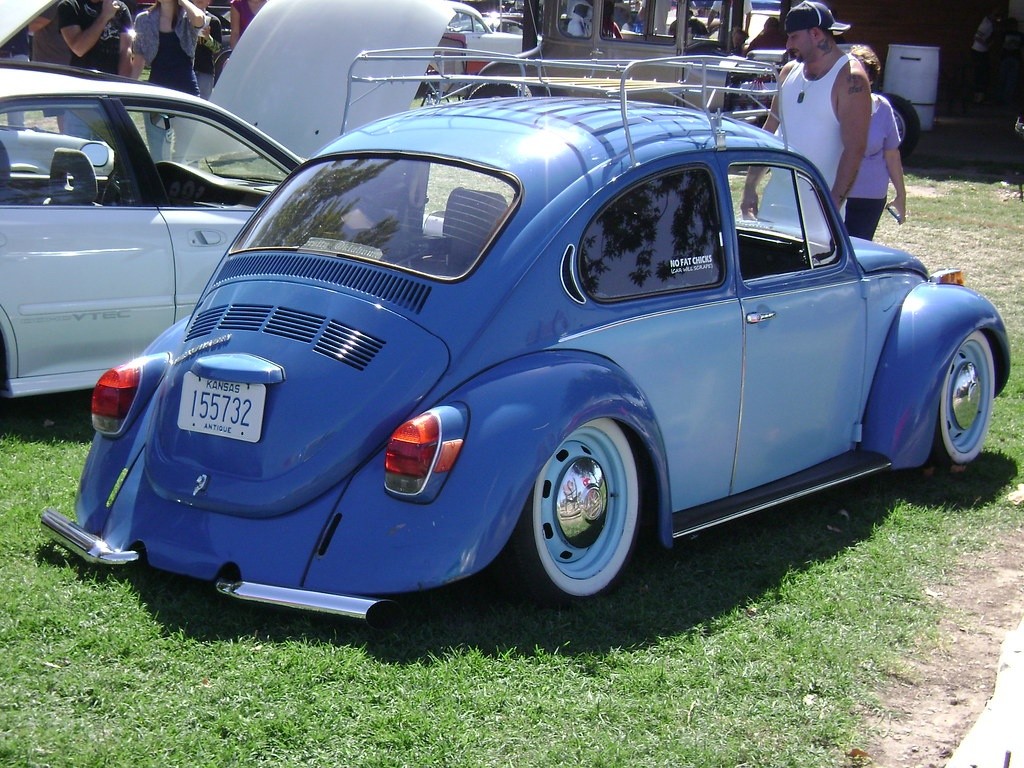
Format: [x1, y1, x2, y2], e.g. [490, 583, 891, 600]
[784, 1, 851, 35]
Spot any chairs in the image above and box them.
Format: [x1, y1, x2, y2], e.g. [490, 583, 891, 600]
[442, 186, 508, 271]
[0, 141, 30, 205]
[43, 147, 103, 207]
[420, 37, 473, 107]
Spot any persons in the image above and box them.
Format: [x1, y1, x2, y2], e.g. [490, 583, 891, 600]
[5, 25, 34, 132]
[565, 0, 709, 41]
[726, 27, 748, 56]
[129, 0, 206, 168]
[744, 17, 788, 59]
[741, 0, 872, 244]
[192, 0, 222, 100]
[56, 0, 134, 142]
[28, 0, 70, 133]
[844, 42, 907, 241]
[960, 0, 1024, 118]
[707, 0, 759, 38]
[230, 0, 269, 53]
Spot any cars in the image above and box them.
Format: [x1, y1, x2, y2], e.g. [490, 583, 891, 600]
[0, 0, 1023, 161]
[39, 54, 1011, 632]
[0, 1, 496, 406]
